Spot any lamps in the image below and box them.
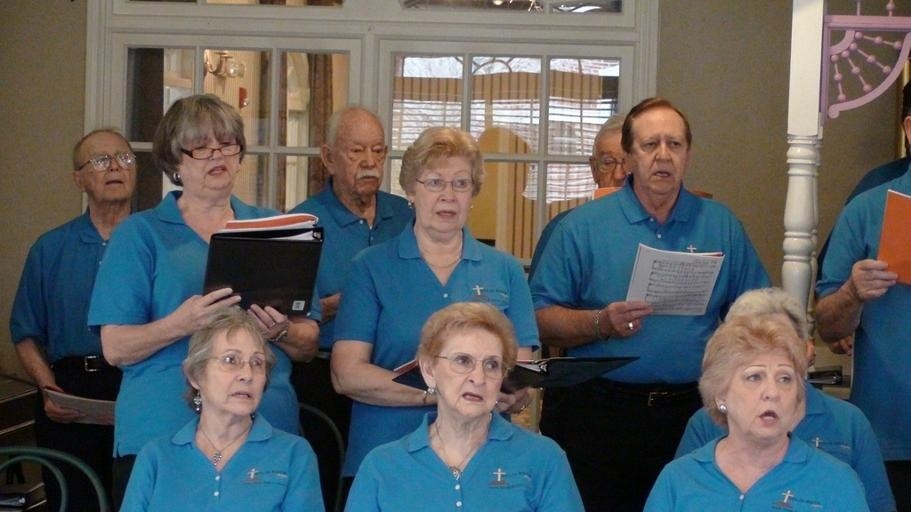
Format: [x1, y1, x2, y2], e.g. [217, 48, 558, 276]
[203, 47, 245, 79]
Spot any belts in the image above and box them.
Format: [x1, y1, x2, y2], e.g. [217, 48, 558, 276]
[645, 388, 667, 404]
[51, 356, 105, 373]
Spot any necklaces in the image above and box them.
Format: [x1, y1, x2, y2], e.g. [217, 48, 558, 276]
[425, 256, 461, 269]
[434, 421, 489, 480]
[198, 422, 252, 465]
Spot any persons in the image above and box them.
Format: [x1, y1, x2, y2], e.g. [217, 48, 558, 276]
[673, 287, 896, 512]
[331, 126, 540, 512]
[119, 308, 326, 512]
[527, 97, 772, 512]
[9, 129, 138, 511]
[813, 84, 910, 512]
[345, 300, 537, 488]
[642, 316, 870, 512]
[285, 105, 417, 512]
[88, 93, 322, 511]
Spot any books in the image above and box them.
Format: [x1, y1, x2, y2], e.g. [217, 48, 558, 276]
[220, 213, 319, 232]
[43, 386, 117, 425]
[394, 356, 575, 374]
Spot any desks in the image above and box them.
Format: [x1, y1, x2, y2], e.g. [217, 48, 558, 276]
[0, 374, 47, 512]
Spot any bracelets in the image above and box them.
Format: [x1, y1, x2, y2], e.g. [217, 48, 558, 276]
[267, 320, 290, 343]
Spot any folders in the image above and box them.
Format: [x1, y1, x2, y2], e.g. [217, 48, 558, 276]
[391, 356, 640, 396]
[202, 227, 326, 320]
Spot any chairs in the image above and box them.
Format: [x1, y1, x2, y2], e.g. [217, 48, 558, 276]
[298, 403, 346, 512]
[0, 446, 109, 512]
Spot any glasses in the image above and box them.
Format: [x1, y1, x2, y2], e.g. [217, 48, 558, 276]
[437, 351, 512, 382]
[414, 176, 477, 194]
[594, 154, 627, 174]
[178, 142, 242, 159]
[203, 349, 270, 375]
[79, 150, 137, 173]
